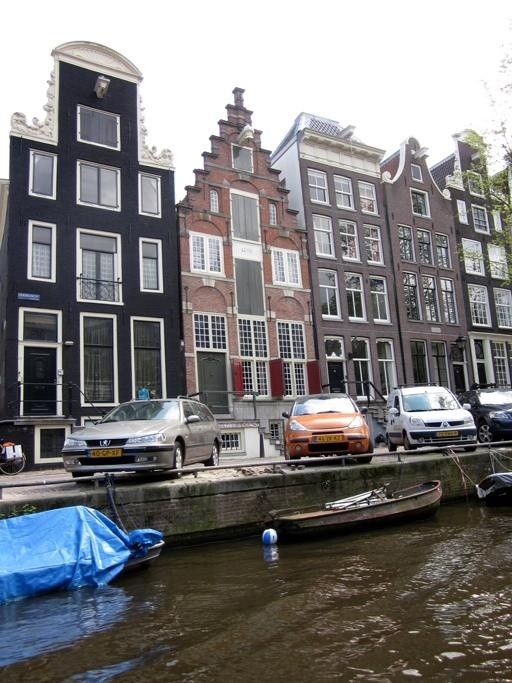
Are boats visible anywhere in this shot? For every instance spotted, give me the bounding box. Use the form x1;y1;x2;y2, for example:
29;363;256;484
264;478;445;538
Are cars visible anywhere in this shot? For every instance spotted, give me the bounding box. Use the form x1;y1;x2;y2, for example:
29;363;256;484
385;385;476;454
282;390;374;466
455;384;511;444
59;397;224;483
